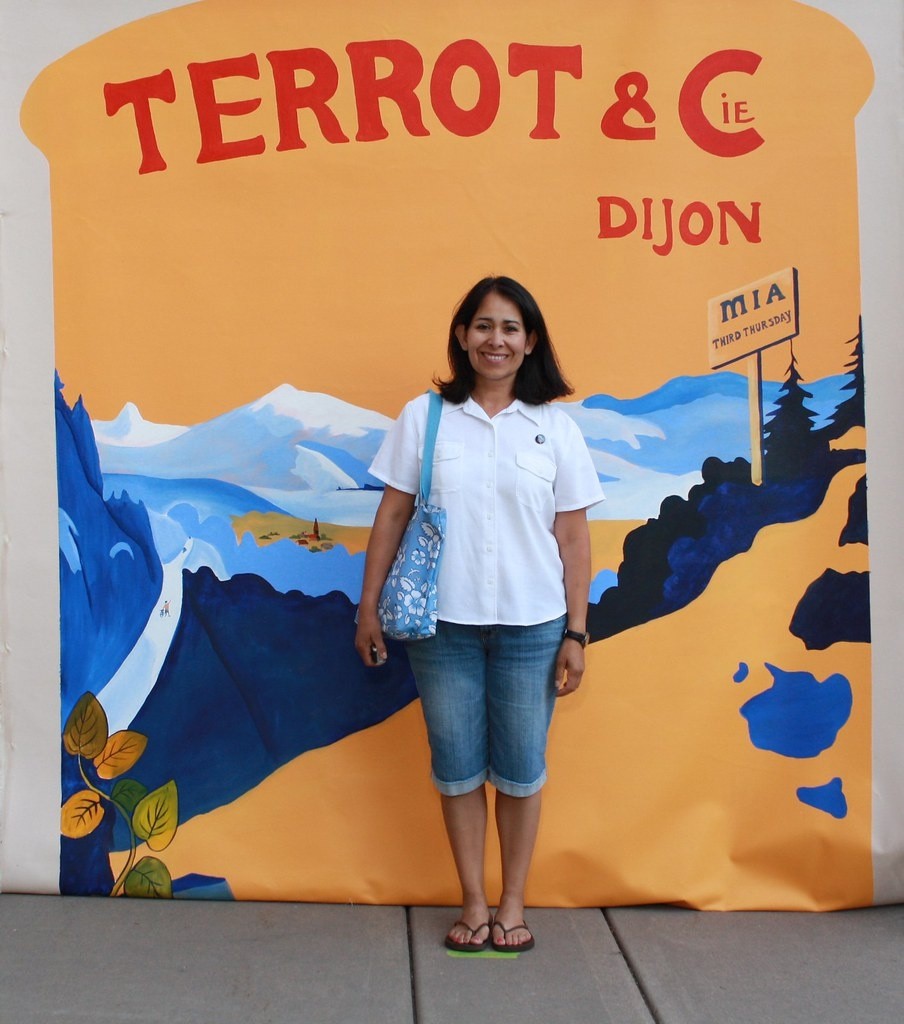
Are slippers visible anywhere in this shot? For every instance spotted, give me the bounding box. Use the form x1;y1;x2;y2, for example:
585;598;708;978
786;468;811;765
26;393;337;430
491;919;535;952
445;910;493;952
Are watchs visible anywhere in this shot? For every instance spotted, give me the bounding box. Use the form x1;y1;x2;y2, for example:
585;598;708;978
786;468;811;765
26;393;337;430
562;626;590;649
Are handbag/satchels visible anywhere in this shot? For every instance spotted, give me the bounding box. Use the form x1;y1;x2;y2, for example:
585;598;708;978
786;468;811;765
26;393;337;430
353;389;449;643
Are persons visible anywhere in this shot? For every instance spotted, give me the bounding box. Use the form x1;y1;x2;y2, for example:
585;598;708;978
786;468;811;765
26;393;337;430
355;274;607;955
